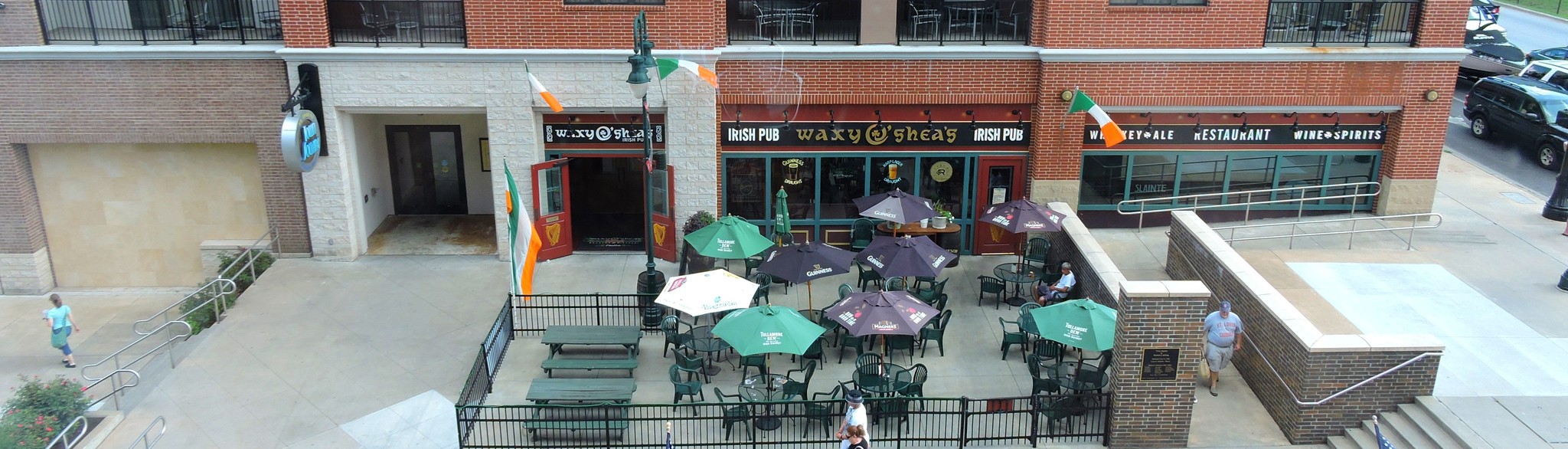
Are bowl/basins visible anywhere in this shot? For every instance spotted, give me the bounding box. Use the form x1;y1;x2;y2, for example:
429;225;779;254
887;219;901;229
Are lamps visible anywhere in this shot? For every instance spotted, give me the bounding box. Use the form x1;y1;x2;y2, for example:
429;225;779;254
563;88;1439;138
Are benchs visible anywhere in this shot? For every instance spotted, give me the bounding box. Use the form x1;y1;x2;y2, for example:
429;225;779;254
541;358;638;370
522;418;628;430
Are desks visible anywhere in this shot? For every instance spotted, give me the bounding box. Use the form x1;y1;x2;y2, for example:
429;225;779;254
1318;20;1347;41
944;0;998;38
682;325;735;376
759;0;812;38
993;262;1044;311
759;242;795;284
395;21;421;41
853;363;912;417
738;373;798;438
220;21;242;39
527;325;641;441
876;221;960;242
1047;361;1110;424
1017;313;1041;350
795;309;838;363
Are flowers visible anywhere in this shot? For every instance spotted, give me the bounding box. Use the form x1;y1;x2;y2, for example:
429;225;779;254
933;199;955;224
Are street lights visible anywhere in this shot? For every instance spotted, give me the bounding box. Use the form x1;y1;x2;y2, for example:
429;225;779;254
626;10;676;327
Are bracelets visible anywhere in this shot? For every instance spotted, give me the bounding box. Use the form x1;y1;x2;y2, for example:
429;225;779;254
840;435;843;440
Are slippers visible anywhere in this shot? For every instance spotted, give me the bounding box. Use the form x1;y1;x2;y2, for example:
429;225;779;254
62;359;68;363
66;362;76;368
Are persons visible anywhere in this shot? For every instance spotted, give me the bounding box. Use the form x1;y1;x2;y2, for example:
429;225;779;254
846;425;869;449
835;389;869;449
992;169;1003;186
43;293;78;368
1033;262;1076;307
1200;301;1245;396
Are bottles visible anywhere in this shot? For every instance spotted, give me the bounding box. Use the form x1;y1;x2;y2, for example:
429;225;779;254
1067;364;1075;376
1012;263;1017;273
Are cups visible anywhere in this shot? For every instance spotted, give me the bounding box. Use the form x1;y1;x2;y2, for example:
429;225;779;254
889;164;897;179
920;218;928;228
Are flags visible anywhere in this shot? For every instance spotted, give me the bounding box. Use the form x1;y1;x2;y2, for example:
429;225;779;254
655;58;718;88
1067;90;1127;148
525;65;564;112
504;160;542;301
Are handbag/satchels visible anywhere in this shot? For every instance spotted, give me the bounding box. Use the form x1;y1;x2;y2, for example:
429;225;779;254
1198;345;1211;379
51;327;67;347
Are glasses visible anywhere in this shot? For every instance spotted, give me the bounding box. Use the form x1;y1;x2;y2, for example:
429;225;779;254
845;433;854;439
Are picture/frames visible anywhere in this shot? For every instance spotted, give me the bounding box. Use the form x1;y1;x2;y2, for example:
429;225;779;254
479;137;491;172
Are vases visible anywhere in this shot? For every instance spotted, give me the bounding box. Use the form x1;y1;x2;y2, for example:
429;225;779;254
931;216;947;229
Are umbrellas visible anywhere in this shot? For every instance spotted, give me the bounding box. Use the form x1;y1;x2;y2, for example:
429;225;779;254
654;269;760;369
773;185;791;247
852;187;940;238
856;235;957;290
682;212;775;271
978;196;1067;271
1029;296;1118;371
756;240;858;320
711;303;827;420
824;289;940;378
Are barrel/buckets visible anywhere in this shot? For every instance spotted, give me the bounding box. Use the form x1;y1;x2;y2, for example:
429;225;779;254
936;229;961;268
637;271;666;315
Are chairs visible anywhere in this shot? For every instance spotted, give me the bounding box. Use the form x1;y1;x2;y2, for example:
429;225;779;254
1267;0;1387;42
358;1;464;40
751;0;1027;41
663;217;1112;442
836;158;962;215
166;0;281;40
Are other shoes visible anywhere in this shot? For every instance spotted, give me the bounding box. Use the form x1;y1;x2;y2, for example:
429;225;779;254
1216;377;1219;382
1209;385;1218;396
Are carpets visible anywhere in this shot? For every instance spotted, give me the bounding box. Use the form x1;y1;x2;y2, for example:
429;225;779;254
576;212;646;251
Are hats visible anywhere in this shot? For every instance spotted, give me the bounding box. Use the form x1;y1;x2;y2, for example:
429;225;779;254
1220;301;1230;312
845;390;864;404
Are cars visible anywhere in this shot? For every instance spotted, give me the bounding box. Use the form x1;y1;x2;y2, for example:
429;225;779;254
1464;0;1508;45
1462;45;1568;170
